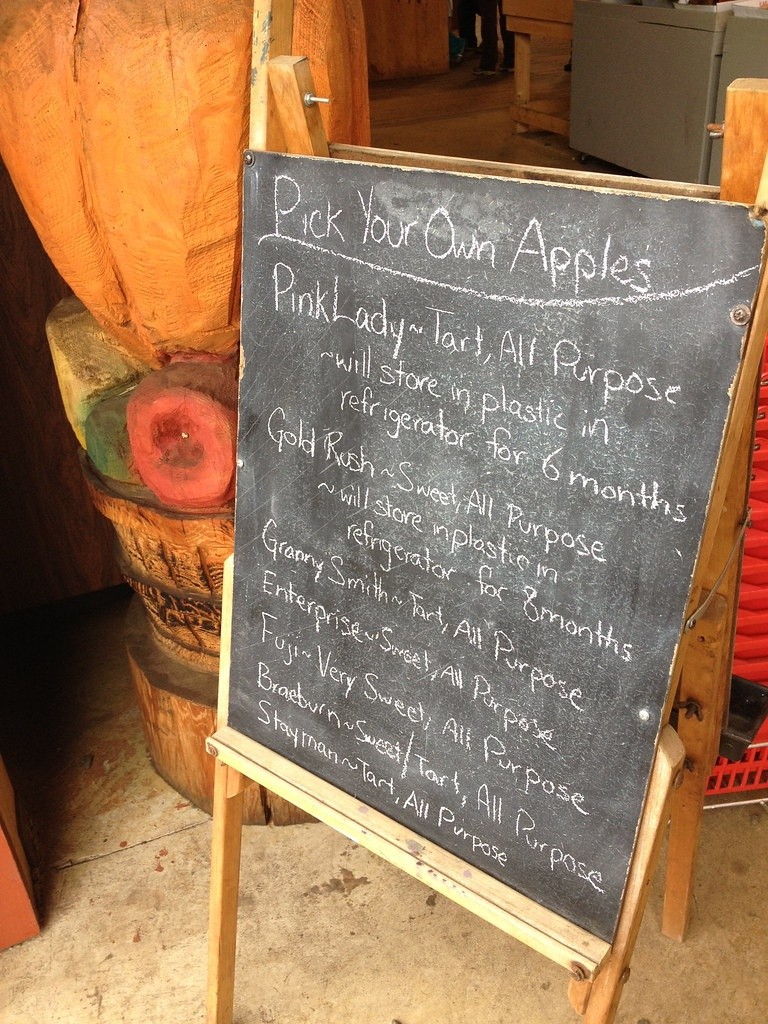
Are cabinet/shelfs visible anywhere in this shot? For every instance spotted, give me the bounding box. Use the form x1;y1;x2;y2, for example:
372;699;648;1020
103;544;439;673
502;0;768;185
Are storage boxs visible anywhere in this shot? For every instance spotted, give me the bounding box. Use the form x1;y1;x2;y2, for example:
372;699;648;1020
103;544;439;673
703;337;767;795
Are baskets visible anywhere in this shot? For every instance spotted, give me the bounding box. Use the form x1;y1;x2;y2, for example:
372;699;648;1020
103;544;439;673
704;339;767;797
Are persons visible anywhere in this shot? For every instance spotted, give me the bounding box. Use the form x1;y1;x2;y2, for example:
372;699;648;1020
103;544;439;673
472;0;516;76
456;0;481;49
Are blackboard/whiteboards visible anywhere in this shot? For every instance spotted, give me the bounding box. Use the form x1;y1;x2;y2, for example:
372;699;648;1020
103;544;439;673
212;145;768;975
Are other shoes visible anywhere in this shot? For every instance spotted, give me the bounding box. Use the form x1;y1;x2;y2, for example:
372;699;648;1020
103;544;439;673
500;63;514;72
472;66;496;76
